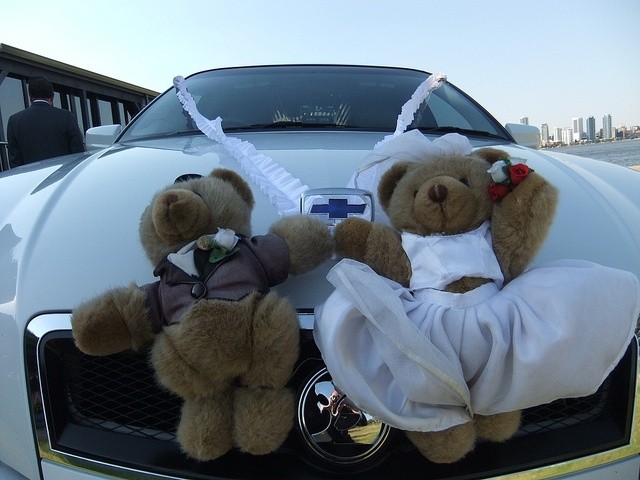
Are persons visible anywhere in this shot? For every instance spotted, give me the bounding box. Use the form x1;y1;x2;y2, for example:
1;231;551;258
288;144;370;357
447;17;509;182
7;75;84;170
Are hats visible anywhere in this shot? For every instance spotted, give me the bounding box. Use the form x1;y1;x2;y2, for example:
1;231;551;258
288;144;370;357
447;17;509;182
29;76;53;98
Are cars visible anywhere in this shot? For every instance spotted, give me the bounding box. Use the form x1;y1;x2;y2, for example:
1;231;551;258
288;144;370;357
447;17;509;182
0;63;640;480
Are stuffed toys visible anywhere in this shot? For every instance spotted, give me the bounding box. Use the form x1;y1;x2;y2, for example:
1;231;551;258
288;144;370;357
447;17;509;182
312;149;640;464
70;169;336;462
